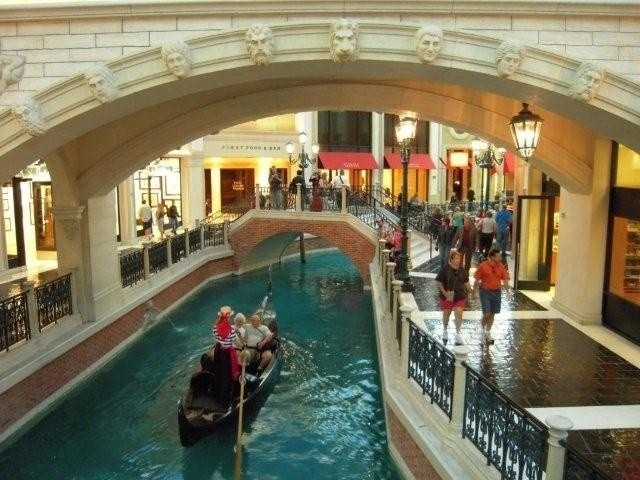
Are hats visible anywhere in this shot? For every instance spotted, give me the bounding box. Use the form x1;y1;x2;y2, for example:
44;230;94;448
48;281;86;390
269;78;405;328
217;306;235;317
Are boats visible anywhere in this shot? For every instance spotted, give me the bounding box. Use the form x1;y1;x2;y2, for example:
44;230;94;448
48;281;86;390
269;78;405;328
177;280;283;448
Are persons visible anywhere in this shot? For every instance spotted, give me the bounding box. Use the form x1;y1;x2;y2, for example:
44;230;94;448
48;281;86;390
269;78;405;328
387;226;402;263
139;199;153;236
156;203;166;240
10;93;48;135
167;199;181;236
568;61;607;105
472;249;511;345
244;21;276;67
493;38;524;80
258;165;351;210
160;38;194;81
187;300;275;414
83;64;119;106
409;188;514;278
377;216;392;249
433;250;472;347
414;25;443;64
325;16;360;64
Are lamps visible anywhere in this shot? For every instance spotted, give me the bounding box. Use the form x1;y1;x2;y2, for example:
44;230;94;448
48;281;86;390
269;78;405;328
506;101;545;165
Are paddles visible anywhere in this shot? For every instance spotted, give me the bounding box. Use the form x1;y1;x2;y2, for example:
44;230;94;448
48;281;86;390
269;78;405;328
234;341;247;480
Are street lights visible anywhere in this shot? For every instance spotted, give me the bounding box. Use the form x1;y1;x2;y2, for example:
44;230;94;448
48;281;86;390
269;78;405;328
471;137;506;216
286;130;320;178
394;111;418;292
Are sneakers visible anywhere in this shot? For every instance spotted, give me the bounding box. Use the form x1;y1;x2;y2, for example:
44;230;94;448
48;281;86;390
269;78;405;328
442;330;495;344
